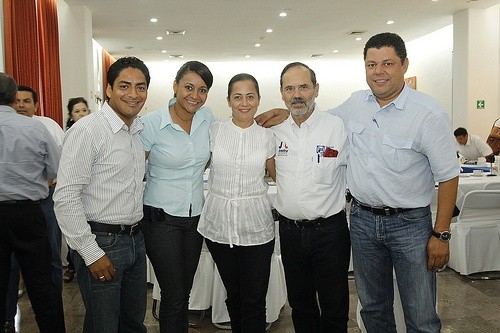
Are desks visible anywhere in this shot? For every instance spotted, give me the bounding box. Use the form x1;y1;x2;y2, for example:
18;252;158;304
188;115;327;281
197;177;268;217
429;172;500;226
146;166;290;284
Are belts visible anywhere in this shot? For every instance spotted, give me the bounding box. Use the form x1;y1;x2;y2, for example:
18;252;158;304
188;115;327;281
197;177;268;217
88;220;139;236
283;216;317;229
352;198;409;217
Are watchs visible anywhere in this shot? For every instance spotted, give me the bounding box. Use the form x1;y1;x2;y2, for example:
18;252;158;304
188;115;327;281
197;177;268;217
432;230;452;242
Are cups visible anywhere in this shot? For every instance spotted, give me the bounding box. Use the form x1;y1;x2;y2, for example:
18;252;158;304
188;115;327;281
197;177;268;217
473;170;483;175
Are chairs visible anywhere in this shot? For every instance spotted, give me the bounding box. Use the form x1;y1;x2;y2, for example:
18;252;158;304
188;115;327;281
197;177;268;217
447;183;500;276
152;190;287;332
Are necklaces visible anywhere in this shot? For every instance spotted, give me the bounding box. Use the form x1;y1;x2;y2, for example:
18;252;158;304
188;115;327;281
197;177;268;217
173;102;193;121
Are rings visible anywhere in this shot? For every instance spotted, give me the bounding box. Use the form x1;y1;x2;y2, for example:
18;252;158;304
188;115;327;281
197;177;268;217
99;276;105;279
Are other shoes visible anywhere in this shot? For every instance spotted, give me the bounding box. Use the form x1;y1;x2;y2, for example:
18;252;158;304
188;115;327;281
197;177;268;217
63;267;74;282
5;319;15;333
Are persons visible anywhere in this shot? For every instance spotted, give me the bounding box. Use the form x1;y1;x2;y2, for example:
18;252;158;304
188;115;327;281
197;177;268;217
53;57;151;333
488;128;500;156
0;72;91;333
197;73;281;333
267;62;349;333
140;61;288;333
454;127;495;163
255;33;459;333
